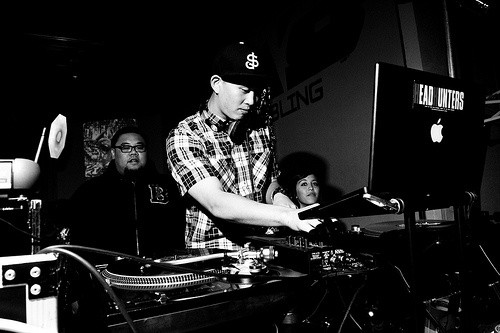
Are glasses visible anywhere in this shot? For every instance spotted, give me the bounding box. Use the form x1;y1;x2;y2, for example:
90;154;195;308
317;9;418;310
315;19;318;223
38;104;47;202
113;144;146;153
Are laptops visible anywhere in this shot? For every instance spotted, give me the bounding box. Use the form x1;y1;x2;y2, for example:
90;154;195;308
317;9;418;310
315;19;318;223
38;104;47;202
298;61;484;220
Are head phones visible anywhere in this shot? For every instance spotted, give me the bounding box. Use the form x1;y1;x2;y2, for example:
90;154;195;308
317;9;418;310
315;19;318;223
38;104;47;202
201;102;248;145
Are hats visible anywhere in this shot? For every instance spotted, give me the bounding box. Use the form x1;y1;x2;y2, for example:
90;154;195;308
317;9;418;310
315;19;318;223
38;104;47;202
212;41;272;88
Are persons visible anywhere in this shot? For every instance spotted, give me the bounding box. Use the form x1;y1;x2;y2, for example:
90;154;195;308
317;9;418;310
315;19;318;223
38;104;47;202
283;162;320;210
69;128;182;263
166;43;338;250
